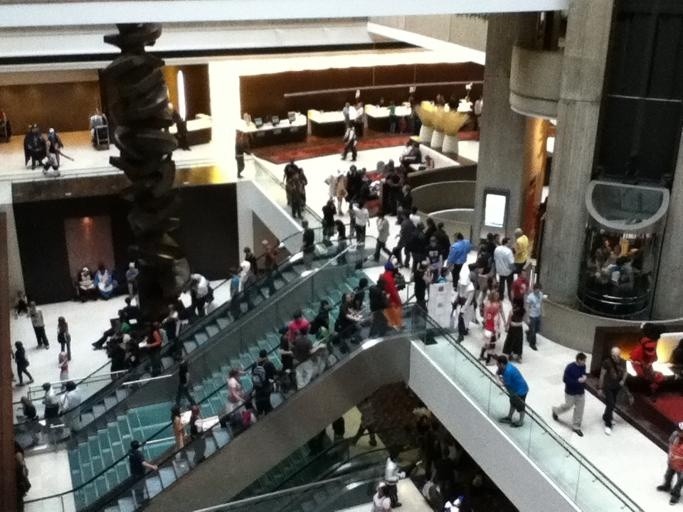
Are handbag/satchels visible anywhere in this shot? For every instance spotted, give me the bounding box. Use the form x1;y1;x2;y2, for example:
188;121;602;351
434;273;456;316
615;386;634;407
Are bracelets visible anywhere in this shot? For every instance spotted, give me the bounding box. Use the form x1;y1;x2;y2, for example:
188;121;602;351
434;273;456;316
596;387;604;390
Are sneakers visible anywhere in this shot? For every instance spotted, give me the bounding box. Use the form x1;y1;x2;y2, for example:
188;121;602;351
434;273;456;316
499;412;615;437
670;496;677;503
454;320;538;366
657;486;668;491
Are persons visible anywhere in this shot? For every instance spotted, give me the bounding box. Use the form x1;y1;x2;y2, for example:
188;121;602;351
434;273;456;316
584;233;655;296
308;394;514;512
321;143;471;345
280;155;308;219
495;354;530;428
655;420;683;505
469;226;546;368
92;215;335;505
337;93;484;162
0;110;12;143
23;122;63;178
551;352;588;437
669;337;683;382
596;345;629;436
630;321;666;394
234;137;251;179
8;266;95;512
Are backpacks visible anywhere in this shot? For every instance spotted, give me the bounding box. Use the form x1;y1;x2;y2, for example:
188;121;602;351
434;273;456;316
251;361;265;388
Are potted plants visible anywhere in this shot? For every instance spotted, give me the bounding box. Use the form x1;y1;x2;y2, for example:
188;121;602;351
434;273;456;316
413;100;470;160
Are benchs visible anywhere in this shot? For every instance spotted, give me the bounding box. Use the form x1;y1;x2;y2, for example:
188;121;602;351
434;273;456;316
399;144;460;171
